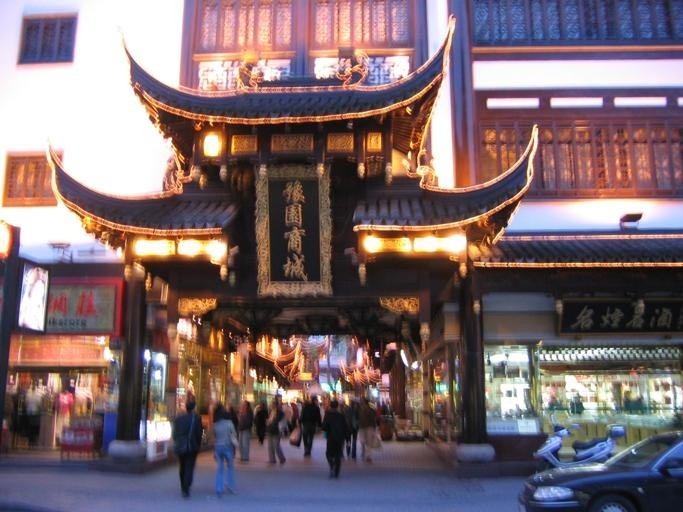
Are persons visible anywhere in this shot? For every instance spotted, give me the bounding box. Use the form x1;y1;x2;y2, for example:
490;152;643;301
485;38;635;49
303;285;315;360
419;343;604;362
621;390;647;414
24;377;75;449
171;391;394;499
549;395;585;415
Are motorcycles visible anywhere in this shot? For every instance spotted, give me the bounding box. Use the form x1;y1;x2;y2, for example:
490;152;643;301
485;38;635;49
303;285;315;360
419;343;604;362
532;414;626;471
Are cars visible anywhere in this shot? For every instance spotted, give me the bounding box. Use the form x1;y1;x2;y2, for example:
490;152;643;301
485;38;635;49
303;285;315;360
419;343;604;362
519;430;683;511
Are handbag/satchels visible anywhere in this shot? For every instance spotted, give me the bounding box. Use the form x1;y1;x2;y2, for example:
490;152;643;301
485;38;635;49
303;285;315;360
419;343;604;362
324;432;327;438
231;434;240;448
371;432;380;448
173;438;192;455
289;426;302;446
